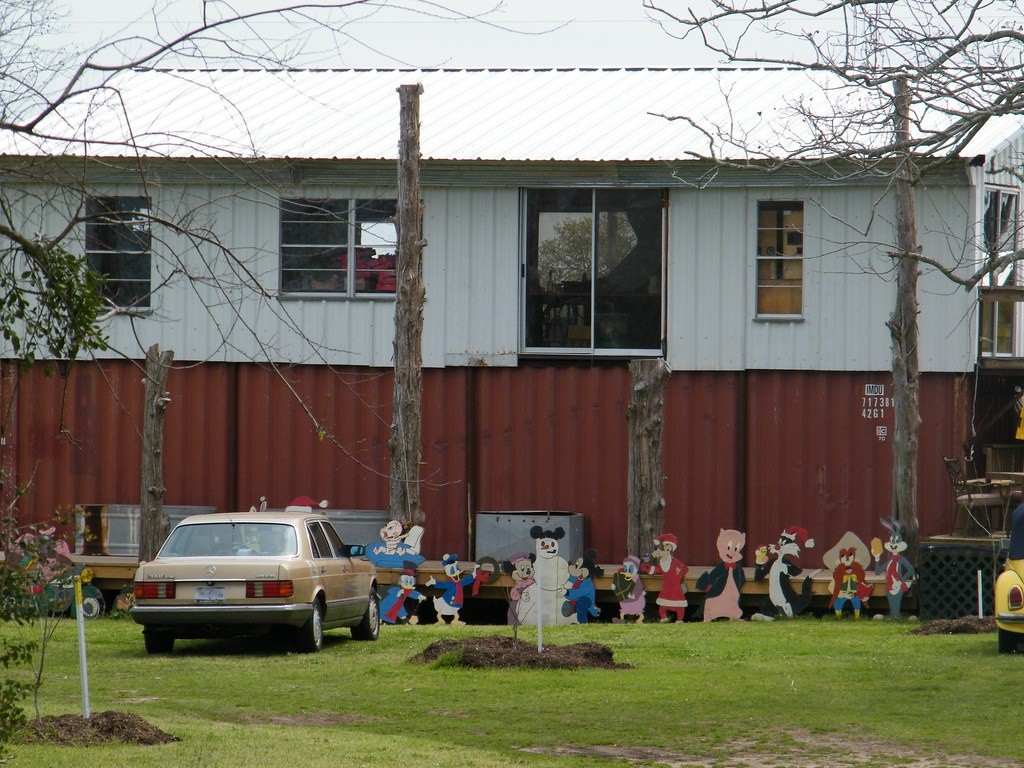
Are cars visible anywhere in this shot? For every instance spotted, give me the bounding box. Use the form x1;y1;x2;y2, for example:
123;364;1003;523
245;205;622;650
994;498;1024;653
130;511;379;652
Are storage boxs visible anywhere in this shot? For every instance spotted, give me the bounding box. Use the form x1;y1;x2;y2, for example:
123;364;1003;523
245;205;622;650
758;210;777;280
476;509;583;565
783;210;803;279
758;280;802;315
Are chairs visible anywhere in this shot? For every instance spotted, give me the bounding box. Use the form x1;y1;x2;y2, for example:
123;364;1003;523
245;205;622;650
942;454;1009;538
